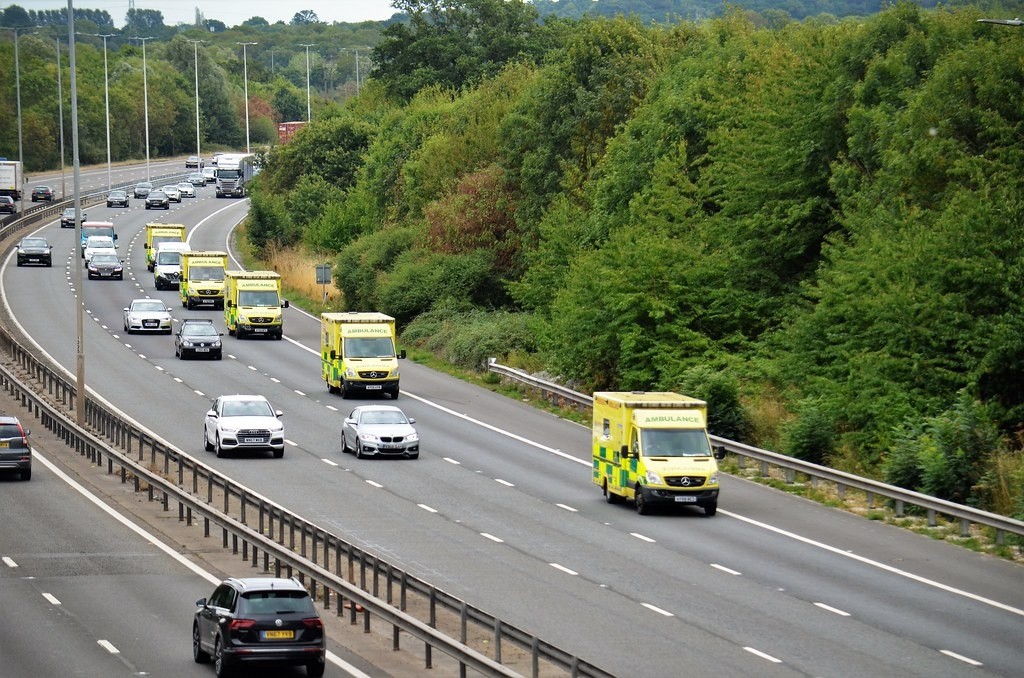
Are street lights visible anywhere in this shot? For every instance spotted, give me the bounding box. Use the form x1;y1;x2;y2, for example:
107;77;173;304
342;47;371;97
47;33;70;204
0;25;43;220
235;41;260;153
126;36;160;183
299;43;317;125
75;31;120;193
184;38;209;174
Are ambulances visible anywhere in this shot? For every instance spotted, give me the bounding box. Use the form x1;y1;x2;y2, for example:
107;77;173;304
320;311;407;400
144;222;187;273
590;392;727;517
180;251;229;311
224;270;290;341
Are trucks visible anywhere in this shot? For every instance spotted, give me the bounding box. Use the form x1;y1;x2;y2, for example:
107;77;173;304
277;122;306;145
215;154;255;199
0;161;29;200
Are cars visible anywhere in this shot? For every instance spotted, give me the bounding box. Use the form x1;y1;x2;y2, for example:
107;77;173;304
82;236;120;269
87;251;125;280
32;186;56;203
186;156;205;168
133;182;154;198
187;173;207;187
159;186;182;203
211;153;224;164
59;208;88;228
106;191;130;208
201;167;217;183
122;299;174;335
177;183;196;198
339;403;421;460
173;319;224;360
145;191;170;210
0;196;17;215
15;237;53;267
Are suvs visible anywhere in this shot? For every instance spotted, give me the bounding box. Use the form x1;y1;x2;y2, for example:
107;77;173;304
202;394;286;458
191;577;325;678
0;417;35;481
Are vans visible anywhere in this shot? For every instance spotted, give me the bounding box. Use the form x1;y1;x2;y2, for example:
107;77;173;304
80;222;118;258
154;241;191;292
